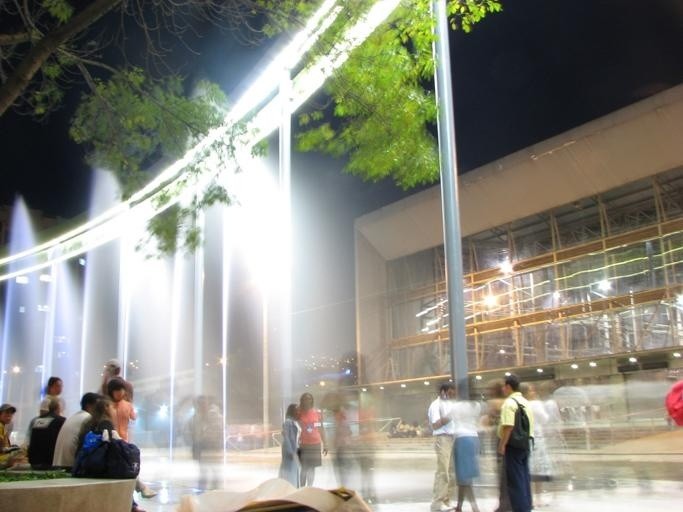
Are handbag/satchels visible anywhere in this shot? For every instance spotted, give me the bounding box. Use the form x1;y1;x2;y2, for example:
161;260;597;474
83;437;140;476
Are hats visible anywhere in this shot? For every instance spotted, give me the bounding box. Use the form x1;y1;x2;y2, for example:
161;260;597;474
0;404;16;413
107;359;120;369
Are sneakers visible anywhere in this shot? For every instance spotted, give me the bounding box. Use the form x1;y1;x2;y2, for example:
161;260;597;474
141;489;157;498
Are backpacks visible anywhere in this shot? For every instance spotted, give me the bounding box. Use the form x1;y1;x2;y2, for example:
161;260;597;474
509;395;530;451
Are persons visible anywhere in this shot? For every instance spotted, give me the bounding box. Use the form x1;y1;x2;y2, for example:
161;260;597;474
278;392;328;488
1;360;158;511
427;373;485;511
479;374;554;511
387;418;433;439
182;394;225;494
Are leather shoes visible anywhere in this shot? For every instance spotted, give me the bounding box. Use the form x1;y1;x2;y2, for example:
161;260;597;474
431;503;454;512
